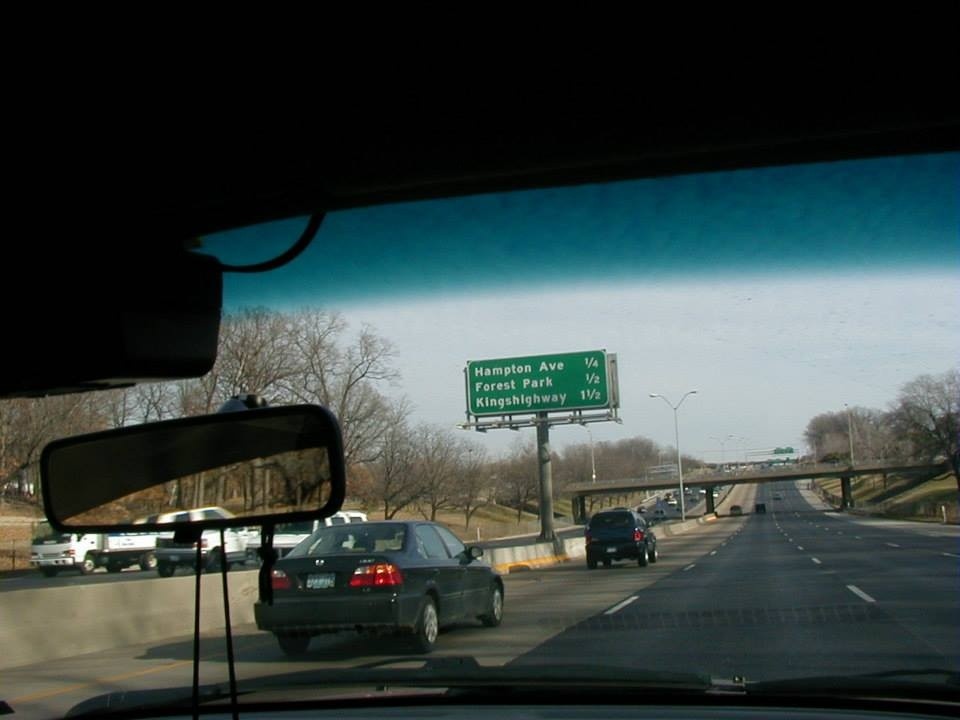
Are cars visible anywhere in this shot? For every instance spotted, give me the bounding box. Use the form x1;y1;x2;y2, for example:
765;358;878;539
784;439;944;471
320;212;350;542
685;489;692;495
668;498;677;504
712;491;718;497
665;493;672;499
699;489;706;495
254;520;504;653
774;491;782;499
730;505;742;515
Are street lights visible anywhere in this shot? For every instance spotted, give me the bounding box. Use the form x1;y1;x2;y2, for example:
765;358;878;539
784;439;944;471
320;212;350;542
649;390;698;522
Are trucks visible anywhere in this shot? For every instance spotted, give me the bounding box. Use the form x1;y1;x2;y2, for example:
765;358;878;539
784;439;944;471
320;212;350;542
29;518;158;576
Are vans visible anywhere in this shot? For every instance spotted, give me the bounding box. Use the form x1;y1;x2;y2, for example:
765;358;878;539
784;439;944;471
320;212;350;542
654;509;665;518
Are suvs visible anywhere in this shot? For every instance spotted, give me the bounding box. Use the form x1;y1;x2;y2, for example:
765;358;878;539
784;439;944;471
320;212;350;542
585;507;658;569
153;525;248;578
756;503;765;512
132;506;367;558
